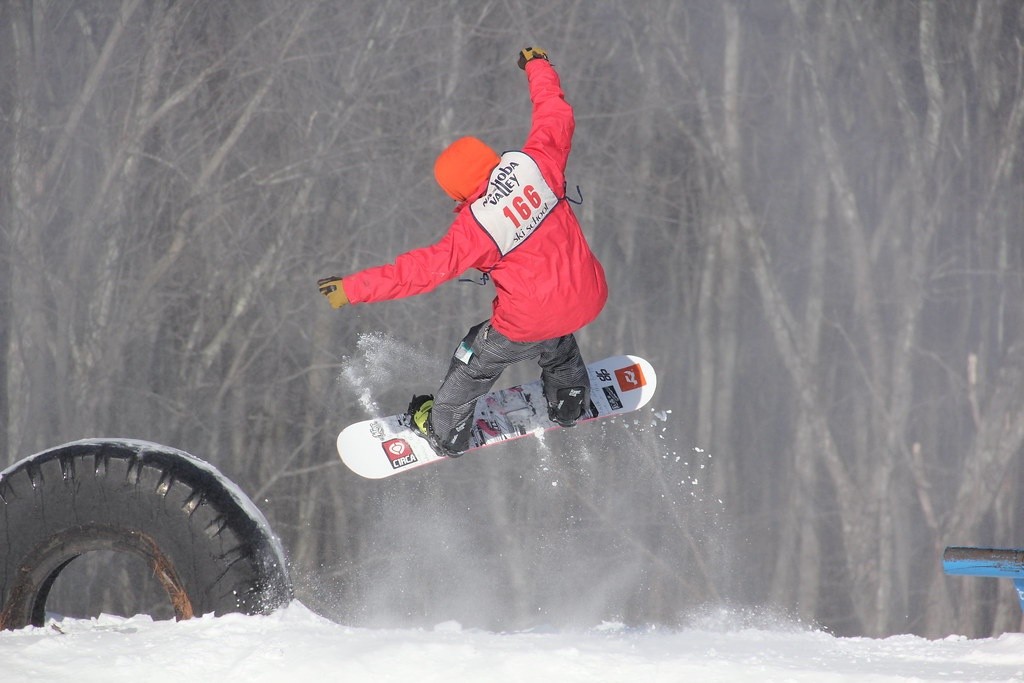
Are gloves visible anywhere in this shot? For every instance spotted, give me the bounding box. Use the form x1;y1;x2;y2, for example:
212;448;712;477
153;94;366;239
517;47;548;69
316;276;347;308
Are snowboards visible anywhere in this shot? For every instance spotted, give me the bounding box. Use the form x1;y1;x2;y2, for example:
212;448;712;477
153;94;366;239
335;354;658;481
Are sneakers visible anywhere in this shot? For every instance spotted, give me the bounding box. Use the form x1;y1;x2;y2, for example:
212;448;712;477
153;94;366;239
540;369;584;427
413;393;463;458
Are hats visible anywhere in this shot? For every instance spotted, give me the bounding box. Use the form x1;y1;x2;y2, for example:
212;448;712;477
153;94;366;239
434;137;500;202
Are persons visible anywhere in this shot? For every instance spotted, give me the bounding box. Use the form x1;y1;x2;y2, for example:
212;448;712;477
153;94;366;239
317;48;609;457
